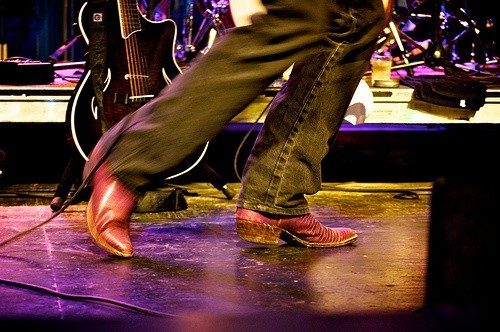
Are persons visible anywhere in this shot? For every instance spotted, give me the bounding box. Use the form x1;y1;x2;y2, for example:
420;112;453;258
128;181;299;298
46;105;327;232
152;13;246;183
82;0;385;258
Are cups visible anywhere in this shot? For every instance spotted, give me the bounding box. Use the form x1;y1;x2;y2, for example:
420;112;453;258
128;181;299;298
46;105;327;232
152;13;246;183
372;55;392;86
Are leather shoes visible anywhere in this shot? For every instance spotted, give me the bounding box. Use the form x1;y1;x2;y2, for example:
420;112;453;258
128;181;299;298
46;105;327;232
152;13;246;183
84;139;137;257
236;207;358;247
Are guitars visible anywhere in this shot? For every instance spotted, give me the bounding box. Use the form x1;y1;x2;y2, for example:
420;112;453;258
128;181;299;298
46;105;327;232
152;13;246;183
65;0;209;180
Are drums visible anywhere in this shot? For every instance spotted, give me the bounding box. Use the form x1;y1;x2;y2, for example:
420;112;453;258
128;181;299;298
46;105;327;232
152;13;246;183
212;0;267;32
